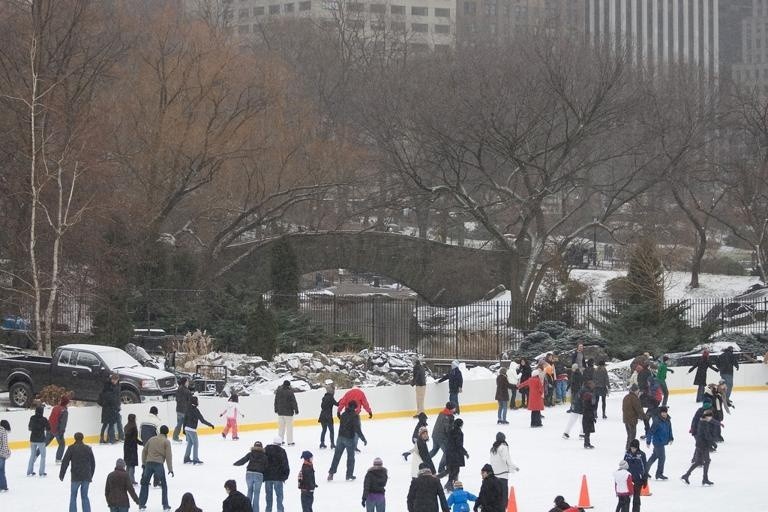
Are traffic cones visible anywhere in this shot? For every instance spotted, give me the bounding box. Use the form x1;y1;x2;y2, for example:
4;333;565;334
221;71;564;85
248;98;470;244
637;475;652;497
506;487;519;512
575;473;596;509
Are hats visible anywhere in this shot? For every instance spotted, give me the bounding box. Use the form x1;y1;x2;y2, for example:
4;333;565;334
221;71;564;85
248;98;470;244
452;360;459;367
61;396;70;406
418;401;505;442
619;460;629;470
374;457;493;489
632;383;638;391
301;451;313;460
532;370;539;375
355;382;361;387
255;442;262;448
150;407;158;414
581;392;593;401
160;425;168;434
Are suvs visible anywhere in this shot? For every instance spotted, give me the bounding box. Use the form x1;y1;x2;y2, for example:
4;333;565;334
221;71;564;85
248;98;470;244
657;343;745;366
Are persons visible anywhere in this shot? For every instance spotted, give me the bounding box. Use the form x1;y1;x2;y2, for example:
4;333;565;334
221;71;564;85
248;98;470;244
621;344;739;485
493;343;611;449
0;420;11;492
27;406;51;476
336;389;373;420
298;451;318;512
274;380;298;446
222;480;254;511
326;399;356;481
549;438;650;512
233;442;268;512
403;357;521;512
36;396;71;464
317;384;339;449
219;393;244;440
361;457;388;512
97;372;214;512
262;442;289;511
59;432;95;512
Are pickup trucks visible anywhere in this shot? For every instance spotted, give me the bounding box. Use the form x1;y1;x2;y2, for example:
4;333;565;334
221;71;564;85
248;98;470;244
0;343;180;404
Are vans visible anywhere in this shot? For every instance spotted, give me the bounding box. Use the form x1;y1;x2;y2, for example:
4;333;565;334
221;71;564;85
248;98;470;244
132;328;169;342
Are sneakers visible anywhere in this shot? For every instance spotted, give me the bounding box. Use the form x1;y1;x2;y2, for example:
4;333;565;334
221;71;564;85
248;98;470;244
320;442;360;480
100;436;203;510
562;415;607;448
648;473;713;484
233;437;239;439
0;460;64;491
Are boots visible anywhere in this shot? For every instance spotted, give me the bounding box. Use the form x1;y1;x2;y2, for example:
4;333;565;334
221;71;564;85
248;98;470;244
402;452;411;461
